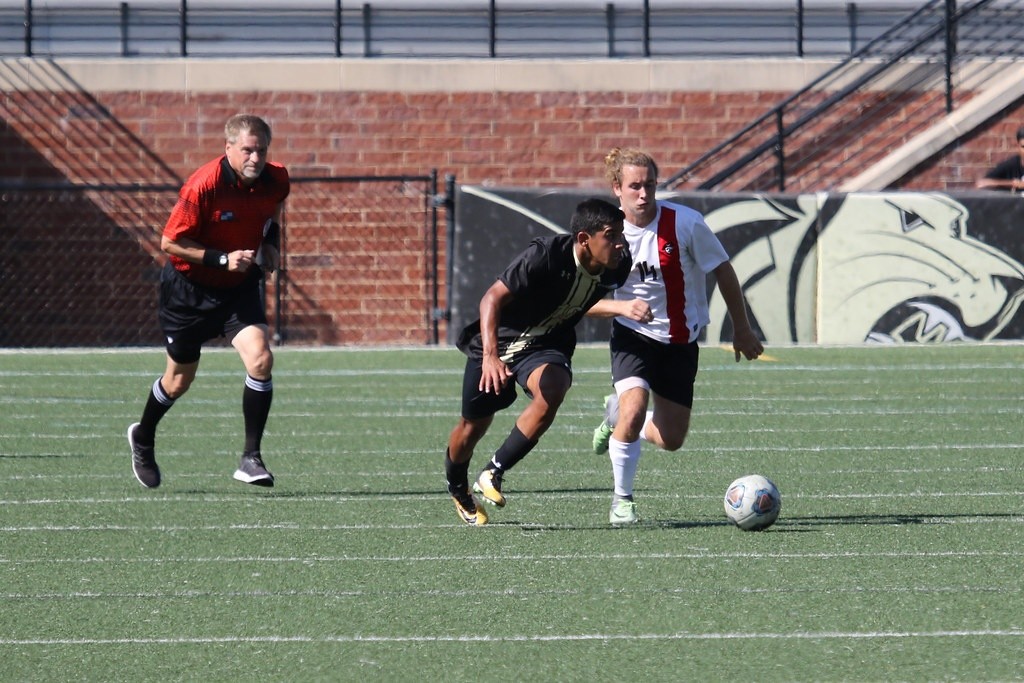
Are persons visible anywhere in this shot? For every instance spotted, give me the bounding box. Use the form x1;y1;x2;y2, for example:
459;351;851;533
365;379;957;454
584;146;764;527
445;198;633;526
127;114;291;490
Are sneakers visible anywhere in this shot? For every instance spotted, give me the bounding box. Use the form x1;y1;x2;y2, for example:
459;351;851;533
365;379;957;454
471;468;506;509
446;481;489;524
232;455;274;487
609;498;639;527
126;422;162;487
592;394;616;456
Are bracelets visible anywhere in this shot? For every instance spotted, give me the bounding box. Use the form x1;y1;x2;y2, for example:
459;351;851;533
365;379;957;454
202;249;228;271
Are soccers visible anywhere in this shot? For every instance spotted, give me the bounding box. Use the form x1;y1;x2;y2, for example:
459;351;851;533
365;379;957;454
724;473;781;531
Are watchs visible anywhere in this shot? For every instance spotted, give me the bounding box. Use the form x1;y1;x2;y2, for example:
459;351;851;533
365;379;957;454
219;252;228;269
